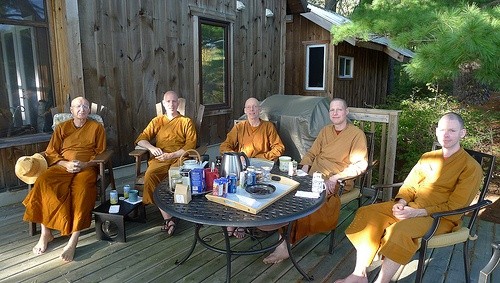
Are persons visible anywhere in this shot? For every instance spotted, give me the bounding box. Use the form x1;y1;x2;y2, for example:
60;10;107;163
220;98;285;239
135;90;198;236
332;112;482;283
22;97;107;263
263;99;368;264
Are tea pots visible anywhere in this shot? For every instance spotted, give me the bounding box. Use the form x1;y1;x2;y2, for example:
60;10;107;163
180;150;209;170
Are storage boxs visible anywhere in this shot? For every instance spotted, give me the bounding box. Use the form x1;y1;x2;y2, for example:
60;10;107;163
180;168;191;191
190;168;206;194
168;167;179;194
173;183;192;205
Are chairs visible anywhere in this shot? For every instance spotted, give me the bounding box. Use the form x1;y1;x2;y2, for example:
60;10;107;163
234;119;279;134
28;103;116;237
330;131;377;257
128;99;206;218
370;141;497;283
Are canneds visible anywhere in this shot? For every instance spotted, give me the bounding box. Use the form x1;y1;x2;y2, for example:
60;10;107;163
123;184;131;198
110;190;118;204
227;166;263;194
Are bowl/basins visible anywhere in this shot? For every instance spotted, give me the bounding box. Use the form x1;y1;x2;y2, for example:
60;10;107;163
245;158;274;172
246;183;276;198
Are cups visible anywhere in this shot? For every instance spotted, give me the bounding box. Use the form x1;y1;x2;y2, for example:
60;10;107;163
205;168;220;188
128;190;138;202
279;156;291;171
311;172;327;193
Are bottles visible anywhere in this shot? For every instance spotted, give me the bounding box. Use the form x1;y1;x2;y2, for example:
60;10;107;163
216;155;222;170
170;175;184;193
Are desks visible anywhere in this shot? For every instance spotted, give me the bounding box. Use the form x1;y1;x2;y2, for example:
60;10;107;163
152;163;326;283
91;194;147;243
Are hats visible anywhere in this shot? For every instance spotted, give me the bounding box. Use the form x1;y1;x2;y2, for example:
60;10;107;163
15;153;49;185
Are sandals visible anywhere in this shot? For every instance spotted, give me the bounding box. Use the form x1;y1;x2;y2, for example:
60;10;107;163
222;227;246;239
160;216;177;236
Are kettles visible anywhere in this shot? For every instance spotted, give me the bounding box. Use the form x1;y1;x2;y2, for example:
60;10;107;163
220;151;250;182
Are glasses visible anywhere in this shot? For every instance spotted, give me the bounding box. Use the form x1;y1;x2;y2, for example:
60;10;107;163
245;104;261;110
329;107;344;113
71;104;89;110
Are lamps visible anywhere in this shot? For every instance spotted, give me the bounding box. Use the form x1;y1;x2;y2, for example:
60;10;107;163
265;8;274;18
235;1;246;11
